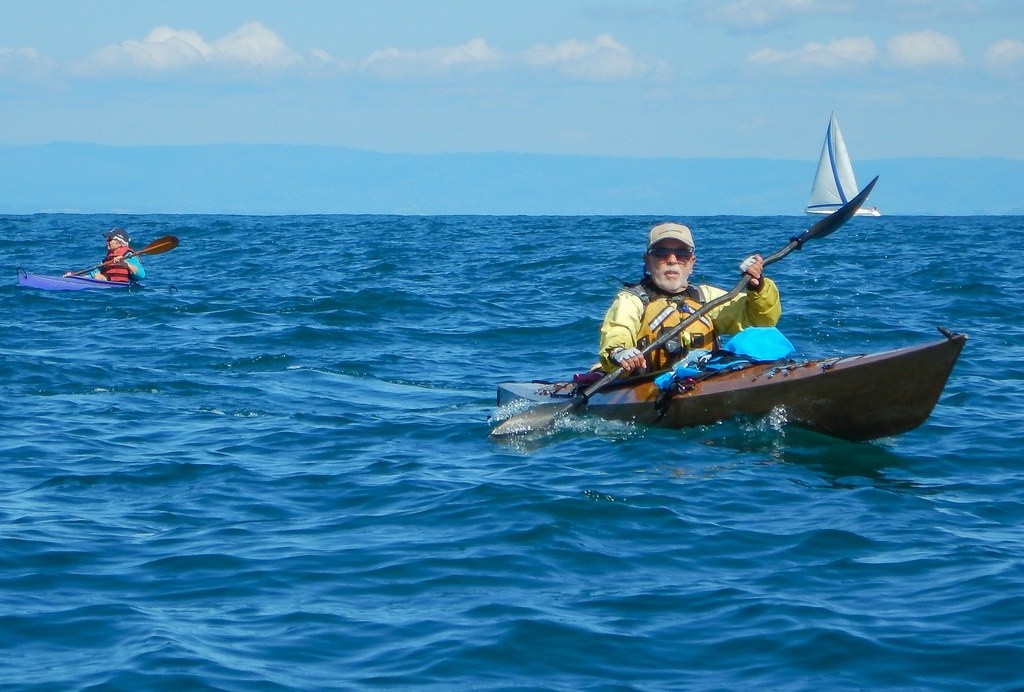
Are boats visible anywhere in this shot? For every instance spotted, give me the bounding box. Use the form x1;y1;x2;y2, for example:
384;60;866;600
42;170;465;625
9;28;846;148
494;324;970;443
16;266;180;296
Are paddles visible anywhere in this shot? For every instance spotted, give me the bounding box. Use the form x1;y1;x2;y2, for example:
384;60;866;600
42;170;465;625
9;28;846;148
489;172;887;439
64;235;181;278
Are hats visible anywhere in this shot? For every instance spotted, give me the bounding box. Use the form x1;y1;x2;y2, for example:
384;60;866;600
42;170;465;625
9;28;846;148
102;229;132;242
649;223;695;248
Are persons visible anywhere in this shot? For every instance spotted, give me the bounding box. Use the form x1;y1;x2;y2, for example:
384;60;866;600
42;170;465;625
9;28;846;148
598;223;783;381
63;228;146;283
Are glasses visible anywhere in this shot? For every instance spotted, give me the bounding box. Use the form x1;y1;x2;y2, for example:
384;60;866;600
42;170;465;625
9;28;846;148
107;238;118;242
646;246;691;259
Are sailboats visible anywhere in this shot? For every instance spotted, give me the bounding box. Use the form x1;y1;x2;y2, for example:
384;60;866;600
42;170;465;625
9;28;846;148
801;106;883;216
